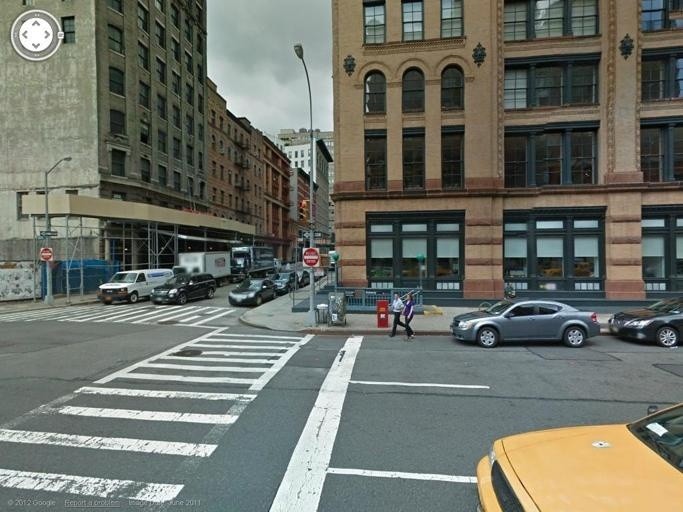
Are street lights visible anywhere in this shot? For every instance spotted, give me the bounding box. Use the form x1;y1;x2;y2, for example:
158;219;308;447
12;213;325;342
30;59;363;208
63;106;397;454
41;154;75;305
292;40;325;331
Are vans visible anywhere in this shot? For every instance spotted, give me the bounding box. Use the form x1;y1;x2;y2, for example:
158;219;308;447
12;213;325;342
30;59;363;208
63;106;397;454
96;267;175;305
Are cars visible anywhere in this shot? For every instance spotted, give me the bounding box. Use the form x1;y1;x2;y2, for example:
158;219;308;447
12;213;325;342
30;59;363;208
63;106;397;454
477;398;682;511
267;267;320;295
447;296;604;349
228;277;278;307
606;296;683;348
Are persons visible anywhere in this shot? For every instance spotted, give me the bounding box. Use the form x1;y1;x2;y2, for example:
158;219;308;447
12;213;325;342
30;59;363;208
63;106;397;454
403;293;414;339
389;291;406;337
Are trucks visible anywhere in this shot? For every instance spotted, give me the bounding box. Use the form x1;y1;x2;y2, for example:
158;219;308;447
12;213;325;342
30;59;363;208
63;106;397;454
170;249;232;289
228;246;275;282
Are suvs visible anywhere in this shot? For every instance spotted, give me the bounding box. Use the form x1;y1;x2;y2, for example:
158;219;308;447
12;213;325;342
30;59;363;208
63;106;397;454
150;272;218;307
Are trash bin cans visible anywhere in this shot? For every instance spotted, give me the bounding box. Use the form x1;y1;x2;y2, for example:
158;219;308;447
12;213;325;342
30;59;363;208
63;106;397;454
316;304;328;323
